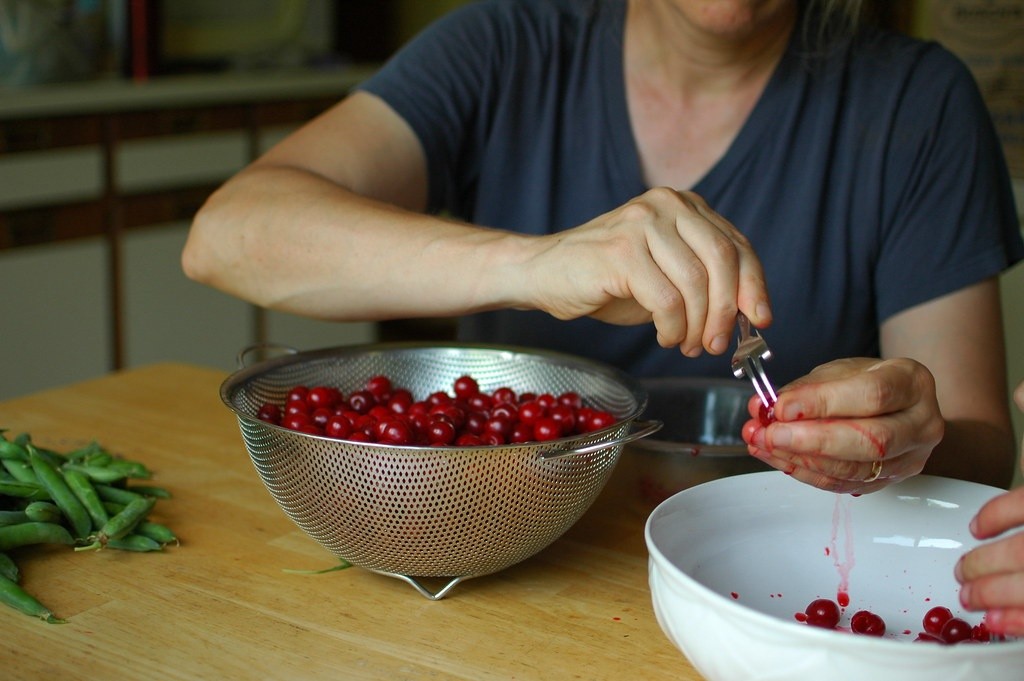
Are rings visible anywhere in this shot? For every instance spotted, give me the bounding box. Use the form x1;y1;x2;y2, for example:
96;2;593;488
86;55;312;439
862;460;884;483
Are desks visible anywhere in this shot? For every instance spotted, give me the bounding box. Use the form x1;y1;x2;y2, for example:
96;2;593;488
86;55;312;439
1;360;746;680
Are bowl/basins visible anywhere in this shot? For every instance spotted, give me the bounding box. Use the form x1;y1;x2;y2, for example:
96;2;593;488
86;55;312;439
622;376;777;520
643;471;1024;681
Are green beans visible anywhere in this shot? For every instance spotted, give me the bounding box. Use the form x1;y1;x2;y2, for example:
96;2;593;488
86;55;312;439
0;424;178;623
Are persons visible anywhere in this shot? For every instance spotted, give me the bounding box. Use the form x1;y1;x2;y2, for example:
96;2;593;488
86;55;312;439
181;0;1024;494
954;381;1024;640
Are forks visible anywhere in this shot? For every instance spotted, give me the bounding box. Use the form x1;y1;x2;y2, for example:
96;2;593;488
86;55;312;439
734;311;780;409
